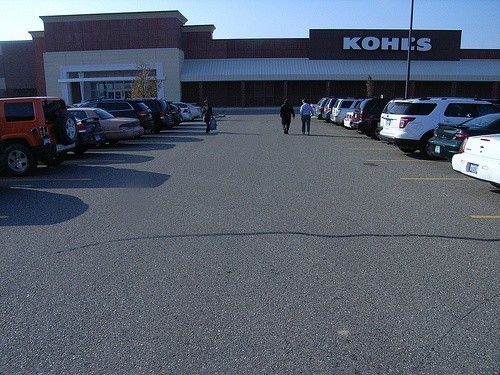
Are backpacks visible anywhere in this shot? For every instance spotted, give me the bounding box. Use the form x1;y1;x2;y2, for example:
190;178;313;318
209;117;216;130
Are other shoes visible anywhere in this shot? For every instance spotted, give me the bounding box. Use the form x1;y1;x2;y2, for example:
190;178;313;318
307;132;309;135
206;129;210;133
284;128;288;134
302;132;305;135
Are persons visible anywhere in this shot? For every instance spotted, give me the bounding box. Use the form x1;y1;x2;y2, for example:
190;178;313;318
300;99;314;136
280;99;295;135
200;98;214;133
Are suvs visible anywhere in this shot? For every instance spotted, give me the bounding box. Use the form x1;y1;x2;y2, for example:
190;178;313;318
73;99;175;139
311;96;500;155
0;98;105;176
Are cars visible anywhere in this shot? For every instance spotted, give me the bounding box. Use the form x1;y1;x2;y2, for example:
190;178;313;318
68;108;145;154
427;113;500;188
168;97;201;133
